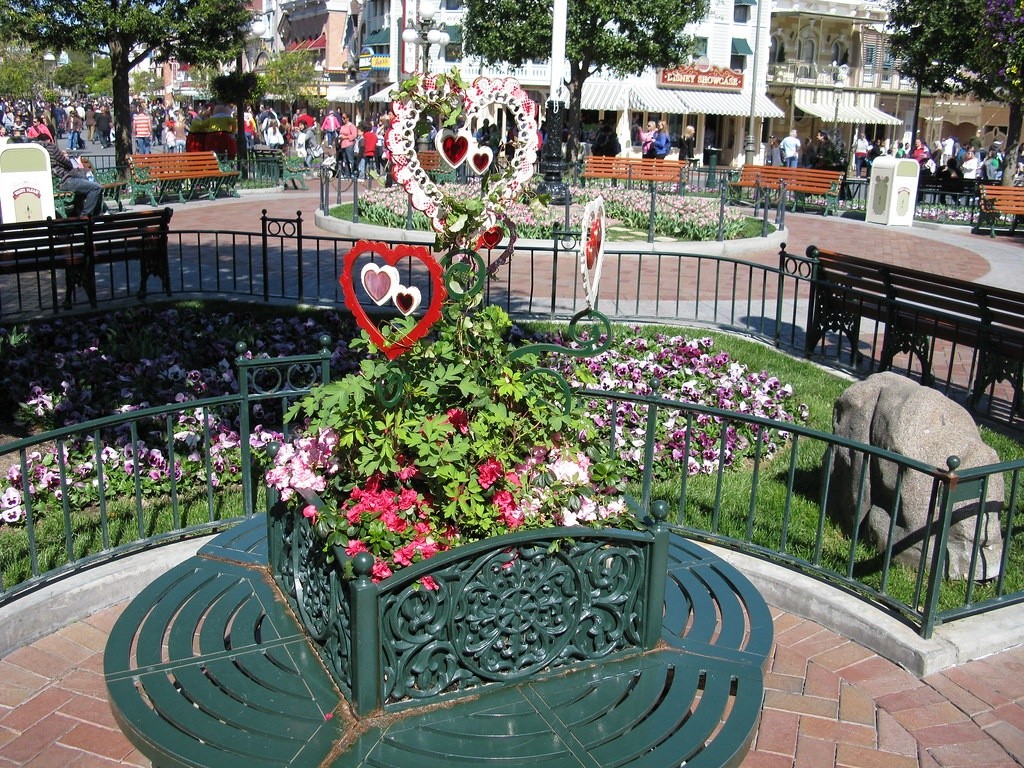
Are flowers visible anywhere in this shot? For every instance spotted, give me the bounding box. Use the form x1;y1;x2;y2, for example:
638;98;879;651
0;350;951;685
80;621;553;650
267;65;645;595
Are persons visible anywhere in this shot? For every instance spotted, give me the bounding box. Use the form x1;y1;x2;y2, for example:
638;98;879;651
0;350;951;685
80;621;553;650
631;114;695;175
852;133;1024;206
35;133;103;221
0;97;437;187
476;118;500;175
506;119;518;168
768;129;837;169
539;120;619;174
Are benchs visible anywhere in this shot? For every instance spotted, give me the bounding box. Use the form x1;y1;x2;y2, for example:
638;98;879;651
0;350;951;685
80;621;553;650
971;184;1024;238
727;164;845;217
411;150;458;185
919;175;1002;204
55;159;130;219
578;156;690;196
126;153;242;208
0;206;173;310
805;245;1024;418
251;147;311;190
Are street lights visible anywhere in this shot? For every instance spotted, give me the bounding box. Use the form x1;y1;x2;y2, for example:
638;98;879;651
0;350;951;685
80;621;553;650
43;51;57;145
402;1;450;151
221;17;266;183
150;77;156;146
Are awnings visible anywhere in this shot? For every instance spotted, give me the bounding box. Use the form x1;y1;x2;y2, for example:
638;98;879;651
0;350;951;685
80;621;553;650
735;0;757;6
368;82;400;104
794;88;904;125
177;61;192;72
732;38;754;56
327;78;368;104
441;25;463;43
361;27;390;46
545;82;785;120
358;53;390;71
263;86;327;101
284;33;325;53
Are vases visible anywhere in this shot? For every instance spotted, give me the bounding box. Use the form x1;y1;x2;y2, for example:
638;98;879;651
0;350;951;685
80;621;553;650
265;441;670;721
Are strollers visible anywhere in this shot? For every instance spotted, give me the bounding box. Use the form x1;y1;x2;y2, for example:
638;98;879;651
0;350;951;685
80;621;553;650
316;138;343;178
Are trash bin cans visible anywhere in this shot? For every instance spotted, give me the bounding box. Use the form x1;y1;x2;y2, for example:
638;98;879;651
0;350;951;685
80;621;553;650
0;143;56;251
866;156;920;225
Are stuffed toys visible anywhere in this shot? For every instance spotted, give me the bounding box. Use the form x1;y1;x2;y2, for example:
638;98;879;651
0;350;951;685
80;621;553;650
186;106;215;199
190;105;237;197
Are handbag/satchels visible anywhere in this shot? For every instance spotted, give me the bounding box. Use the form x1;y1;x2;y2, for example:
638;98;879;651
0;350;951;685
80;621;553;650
310;145;324;158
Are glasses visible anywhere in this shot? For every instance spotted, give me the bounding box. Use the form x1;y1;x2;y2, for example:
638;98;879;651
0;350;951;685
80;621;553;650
647;125;651;127
342;117;349;120
33;121;37;123
